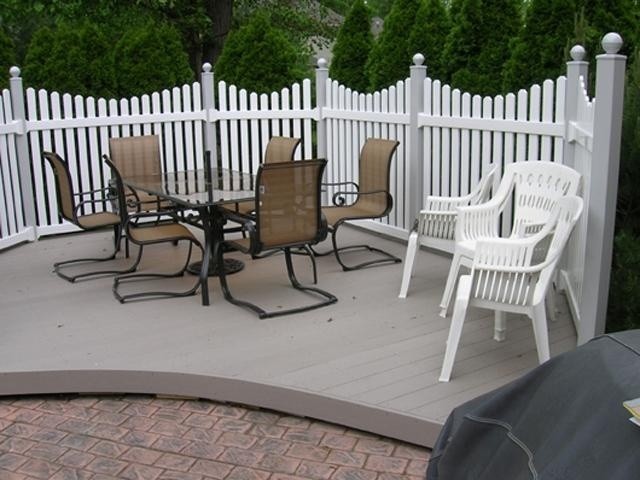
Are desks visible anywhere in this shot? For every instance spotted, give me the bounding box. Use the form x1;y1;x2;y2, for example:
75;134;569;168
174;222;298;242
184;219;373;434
122;167;262;275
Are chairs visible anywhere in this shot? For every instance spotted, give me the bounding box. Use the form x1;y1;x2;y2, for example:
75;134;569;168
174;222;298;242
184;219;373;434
103;134;182;251
320;137;403;270
399;162;499;299
215;159;339;319
439;194;584;381
242;136;303;260
42;151;121;283
101;153;205;302
439;161;582;322
